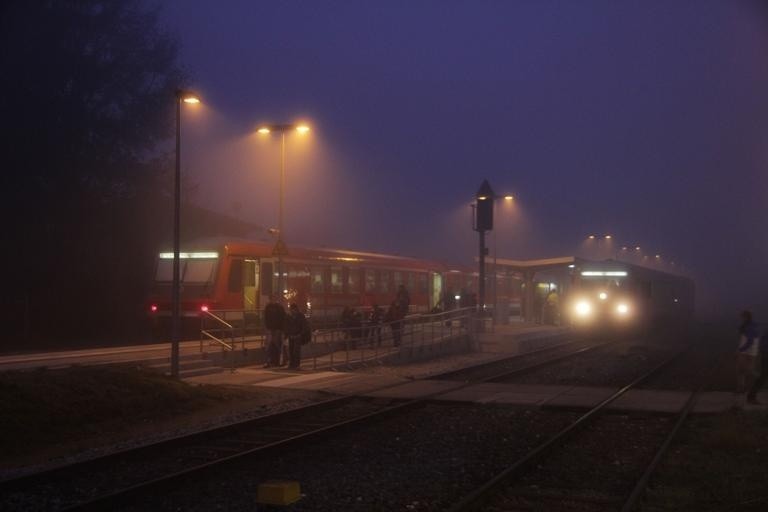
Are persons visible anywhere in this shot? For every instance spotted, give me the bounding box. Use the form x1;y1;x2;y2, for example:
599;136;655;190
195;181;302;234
746;329;768;405
257;284;564;373
733;310;759;394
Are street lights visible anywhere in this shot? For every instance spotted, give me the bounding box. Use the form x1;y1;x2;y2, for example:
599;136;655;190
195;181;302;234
480;193;516;319
256;124;311;302
171;89;203;377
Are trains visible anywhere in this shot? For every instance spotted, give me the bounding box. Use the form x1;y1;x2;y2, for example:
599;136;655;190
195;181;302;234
150;240;526;332
574;258;696;343
